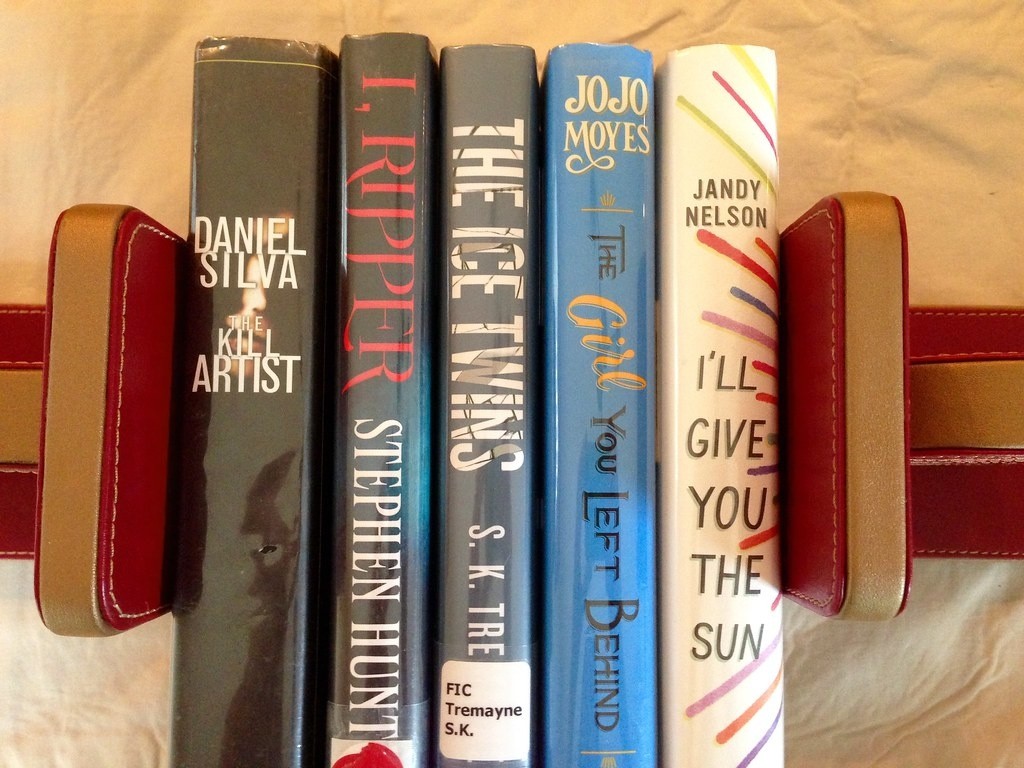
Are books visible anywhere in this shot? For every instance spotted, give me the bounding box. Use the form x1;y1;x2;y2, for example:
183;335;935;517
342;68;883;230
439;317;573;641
433;44;541;768
656;42;785;768
324;32;439;768
168;35;337;768
538;40;660;767
